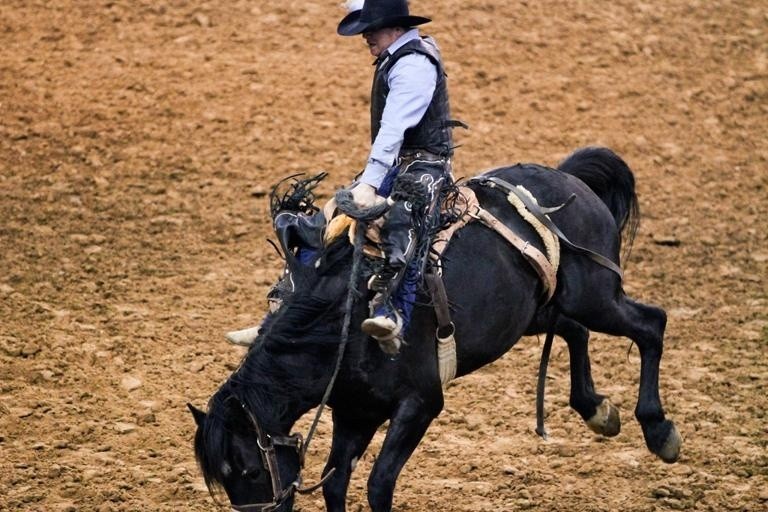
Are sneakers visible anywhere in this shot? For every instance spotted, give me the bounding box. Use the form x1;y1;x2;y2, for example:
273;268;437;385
224;326;261;347
360;315;402;355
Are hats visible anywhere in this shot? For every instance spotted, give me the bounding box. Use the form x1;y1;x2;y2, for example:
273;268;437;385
337;1;432;35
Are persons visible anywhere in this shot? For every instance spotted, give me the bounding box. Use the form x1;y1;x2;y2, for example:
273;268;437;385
226;0;454;357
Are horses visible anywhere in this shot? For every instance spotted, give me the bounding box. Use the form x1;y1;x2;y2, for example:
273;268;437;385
186;147;683;512
